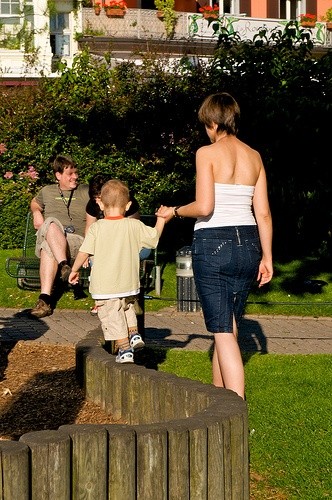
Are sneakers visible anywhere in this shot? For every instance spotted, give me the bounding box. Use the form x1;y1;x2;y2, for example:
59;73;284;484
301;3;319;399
129;333;145;352
61;264;72;281
115;347;134;364
29;299;53;318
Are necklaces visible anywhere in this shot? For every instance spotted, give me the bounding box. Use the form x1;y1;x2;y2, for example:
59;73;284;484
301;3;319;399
217;135;228;142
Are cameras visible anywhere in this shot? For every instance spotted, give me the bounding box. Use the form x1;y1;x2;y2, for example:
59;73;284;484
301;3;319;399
65;225;75;233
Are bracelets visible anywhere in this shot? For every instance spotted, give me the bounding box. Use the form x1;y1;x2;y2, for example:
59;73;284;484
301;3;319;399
173;205;185;220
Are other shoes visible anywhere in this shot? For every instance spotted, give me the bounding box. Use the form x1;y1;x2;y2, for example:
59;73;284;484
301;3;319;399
91;307;98;316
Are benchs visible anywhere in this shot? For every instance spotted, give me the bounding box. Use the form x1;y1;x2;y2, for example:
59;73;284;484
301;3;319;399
5;212;157;295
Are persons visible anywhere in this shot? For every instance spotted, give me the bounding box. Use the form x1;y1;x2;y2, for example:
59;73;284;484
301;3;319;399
155;92;274;401
27;154;90;318
66;172;169;363
